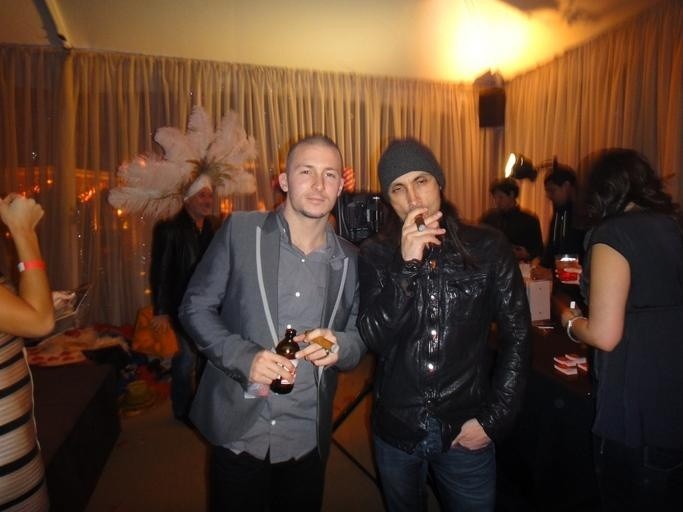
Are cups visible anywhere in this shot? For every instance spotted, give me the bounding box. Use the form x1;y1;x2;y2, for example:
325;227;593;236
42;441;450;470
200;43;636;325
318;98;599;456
554;256;579;282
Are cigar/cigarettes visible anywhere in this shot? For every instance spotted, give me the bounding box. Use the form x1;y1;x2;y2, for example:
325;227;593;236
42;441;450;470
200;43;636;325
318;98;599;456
302;329;341;355
413;212;427;235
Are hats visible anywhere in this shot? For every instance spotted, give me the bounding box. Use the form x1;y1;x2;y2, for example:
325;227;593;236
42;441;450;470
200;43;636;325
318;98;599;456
377;137;447;203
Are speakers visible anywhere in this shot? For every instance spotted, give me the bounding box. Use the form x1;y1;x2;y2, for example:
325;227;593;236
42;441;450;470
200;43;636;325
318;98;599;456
479;86;507;129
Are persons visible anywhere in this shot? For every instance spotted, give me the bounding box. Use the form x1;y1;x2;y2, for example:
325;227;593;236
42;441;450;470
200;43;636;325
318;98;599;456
476;176;544;268
178;131;370;511
147;172;223;432
552;145;683;511
353;135;533;512
0;188;76;511
529;161;591;376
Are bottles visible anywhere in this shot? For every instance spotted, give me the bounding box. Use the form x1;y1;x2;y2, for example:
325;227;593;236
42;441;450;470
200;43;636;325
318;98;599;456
268;328;301;394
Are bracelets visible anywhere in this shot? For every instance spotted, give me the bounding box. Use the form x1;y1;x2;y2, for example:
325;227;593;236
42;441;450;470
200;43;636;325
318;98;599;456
16;259;46;275
566;314;589;344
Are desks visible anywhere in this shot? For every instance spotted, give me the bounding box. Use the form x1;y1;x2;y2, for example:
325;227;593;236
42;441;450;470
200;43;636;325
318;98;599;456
489;317;599;432
